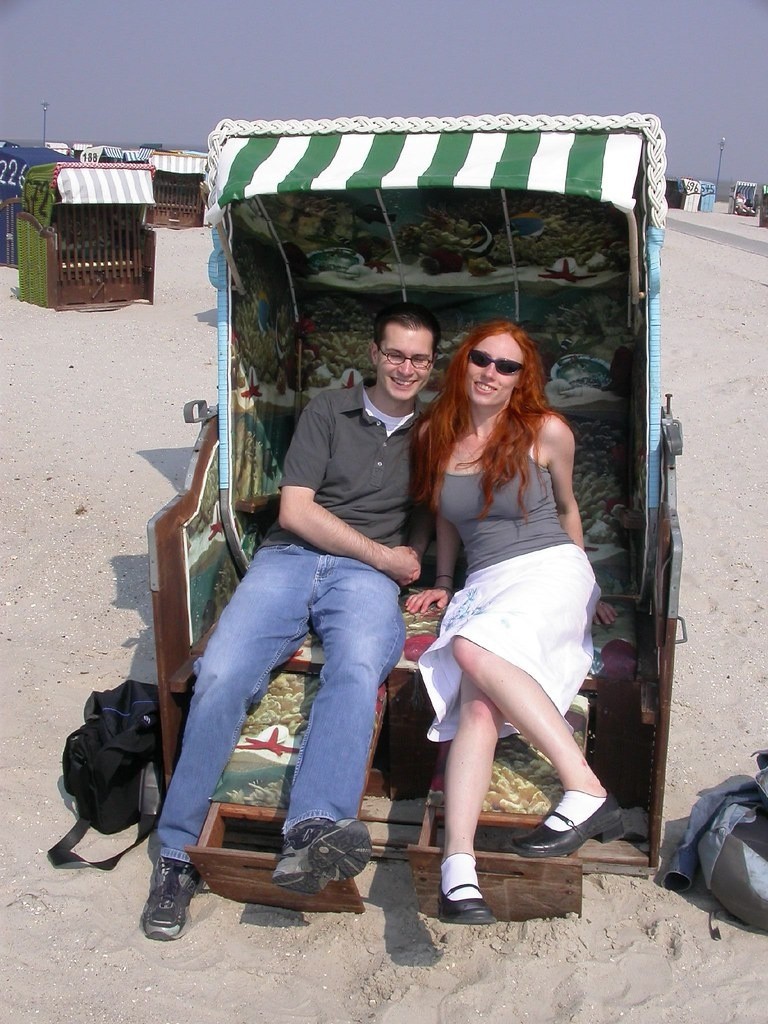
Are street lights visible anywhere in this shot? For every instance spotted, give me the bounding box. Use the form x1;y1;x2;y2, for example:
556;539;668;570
714;136;726;203
41;99;50;147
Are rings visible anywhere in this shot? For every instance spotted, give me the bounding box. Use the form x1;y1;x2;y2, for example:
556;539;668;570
600;602;605;606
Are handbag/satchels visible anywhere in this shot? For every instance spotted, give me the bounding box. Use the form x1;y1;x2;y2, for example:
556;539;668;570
62;680;167;835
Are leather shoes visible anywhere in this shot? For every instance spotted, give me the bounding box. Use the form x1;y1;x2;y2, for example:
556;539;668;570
509;788;625;857
438;877;496;924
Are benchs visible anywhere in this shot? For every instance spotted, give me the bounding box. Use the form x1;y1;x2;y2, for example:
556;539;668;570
143;392;684;925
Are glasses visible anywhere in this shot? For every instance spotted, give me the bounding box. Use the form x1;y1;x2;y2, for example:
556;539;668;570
377;346;433;369
469;350;523;375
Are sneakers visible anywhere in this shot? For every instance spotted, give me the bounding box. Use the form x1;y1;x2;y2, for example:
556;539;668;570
272;818;373;896
143;856;201;941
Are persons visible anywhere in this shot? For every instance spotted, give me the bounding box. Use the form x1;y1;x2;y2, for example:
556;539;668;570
406;320;624;925
143;304;442;941
734;193;755;215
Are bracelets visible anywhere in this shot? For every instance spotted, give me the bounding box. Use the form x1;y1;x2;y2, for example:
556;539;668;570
435;575;453;579
